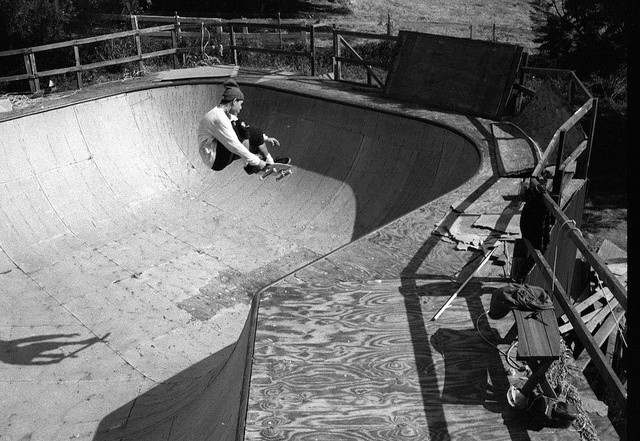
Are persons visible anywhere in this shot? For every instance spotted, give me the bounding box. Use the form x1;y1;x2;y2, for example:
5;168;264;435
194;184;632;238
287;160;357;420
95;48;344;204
198;80;291;175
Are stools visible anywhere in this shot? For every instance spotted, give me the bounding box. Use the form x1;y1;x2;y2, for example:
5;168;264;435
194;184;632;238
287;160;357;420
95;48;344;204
503;286;564;410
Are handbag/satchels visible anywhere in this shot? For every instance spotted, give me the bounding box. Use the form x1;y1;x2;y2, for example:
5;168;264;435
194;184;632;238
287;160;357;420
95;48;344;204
489;284;554;319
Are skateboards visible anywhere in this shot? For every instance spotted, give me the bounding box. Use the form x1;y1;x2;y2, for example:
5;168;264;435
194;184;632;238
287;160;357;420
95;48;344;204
259;163;292;182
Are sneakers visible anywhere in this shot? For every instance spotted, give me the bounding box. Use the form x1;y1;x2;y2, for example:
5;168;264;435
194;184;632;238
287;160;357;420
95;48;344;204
274;157;292;164
244;153;266;175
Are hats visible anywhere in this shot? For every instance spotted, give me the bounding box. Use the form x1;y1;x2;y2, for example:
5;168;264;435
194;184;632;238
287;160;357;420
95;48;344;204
223;79;244;100
